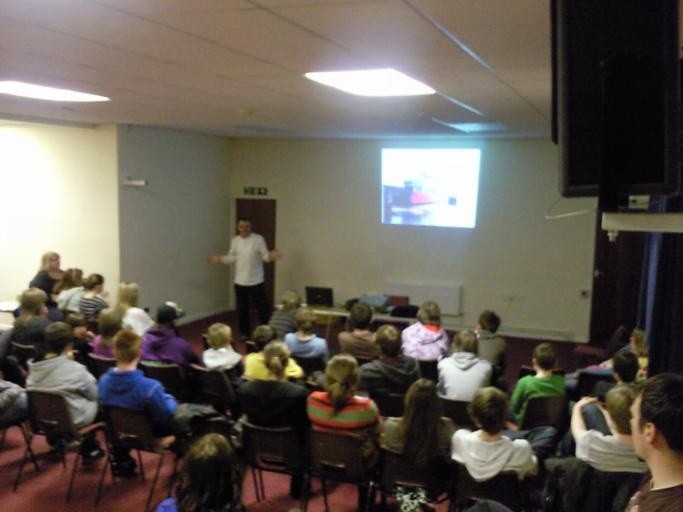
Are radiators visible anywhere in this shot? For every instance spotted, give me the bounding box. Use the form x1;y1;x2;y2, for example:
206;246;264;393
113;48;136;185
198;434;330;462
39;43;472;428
382;280;463;317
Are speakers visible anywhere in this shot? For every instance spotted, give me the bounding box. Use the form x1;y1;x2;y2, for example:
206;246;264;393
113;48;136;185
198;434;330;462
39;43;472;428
556;0;681;198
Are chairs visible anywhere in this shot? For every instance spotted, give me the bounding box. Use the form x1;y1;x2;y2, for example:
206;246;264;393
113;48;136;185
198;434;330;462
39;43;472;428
138;362;180;392
94;402;179;512
189;363;234;410
519;396;564;430
374;389;404;419
0;421;43;478
240;422;305;502
377;445;448;512
291;353;326;379
308;426;376;512
417;357;439;381
201;334;236;350
88;352;116;378
374;293;409;331
437;393;471;427
9;341;36;365
513;366;565;381
12;389;105;502
575;370;613;400
245;339;258;352
355;354;372;367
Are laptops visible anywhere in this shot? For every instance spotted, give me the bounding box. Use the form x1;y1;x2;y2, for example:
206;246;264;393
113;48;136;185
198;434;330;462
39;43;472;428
305;286;333;308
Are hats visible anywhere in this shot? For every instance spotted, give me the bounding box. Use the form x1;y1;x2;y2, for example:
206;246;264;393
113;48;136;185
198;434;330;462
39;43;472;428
156;301;185;323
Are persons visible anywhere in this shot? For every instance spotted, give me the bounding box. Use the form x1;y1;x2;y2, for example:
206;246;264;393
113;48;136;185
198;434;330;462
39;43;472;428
115;281;158;340
383;379;455;454
285;309;328;365
156;433;245;512
600;327;648;382
623;373;683;512
570;387;650;473
475;311;510;393
97;330;178;479
509;344;565;436
306;354;382;511
606;350;646;436
202;323;243;392
11;287;51;353
401;300;449;362
94;307;125;359
207;217;284;336
29;252;64;309
269;290;302;341
435;329;493;403
53;268;84;313
25;322;137;477
360;325;422;394
240;341;314;501
64;313;96;367
338;302;380;359
80;274;109;328
451;387;558;491
141;301;206;366
244;326;304;382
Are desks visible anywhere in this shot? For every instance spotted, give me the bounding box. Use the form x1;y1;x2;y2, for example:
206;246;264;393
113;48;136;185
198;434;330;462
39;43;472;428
274;302;573;343
